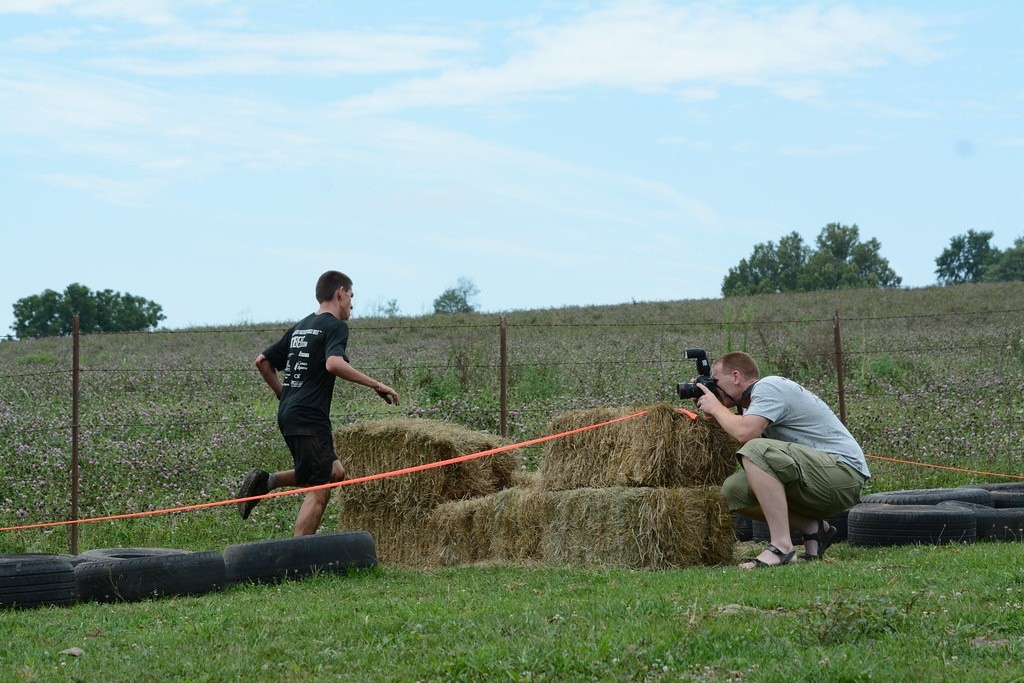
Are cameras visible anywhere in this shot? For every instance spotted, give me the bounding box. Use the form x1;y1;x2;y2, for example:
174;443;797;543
676;349;717;399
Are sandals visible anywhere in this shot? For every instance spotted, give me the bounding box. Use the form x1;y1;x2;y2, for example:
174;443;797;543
744;543;799;569
799;518;838;561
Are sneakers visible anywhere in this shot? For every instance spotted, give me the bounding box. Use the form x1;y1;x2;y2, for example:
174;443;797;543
237;467;270;520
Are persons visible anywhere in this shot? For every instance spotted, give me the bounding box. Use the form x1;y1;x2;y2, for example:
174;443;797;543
238;271;401;537
687;352;871;569
